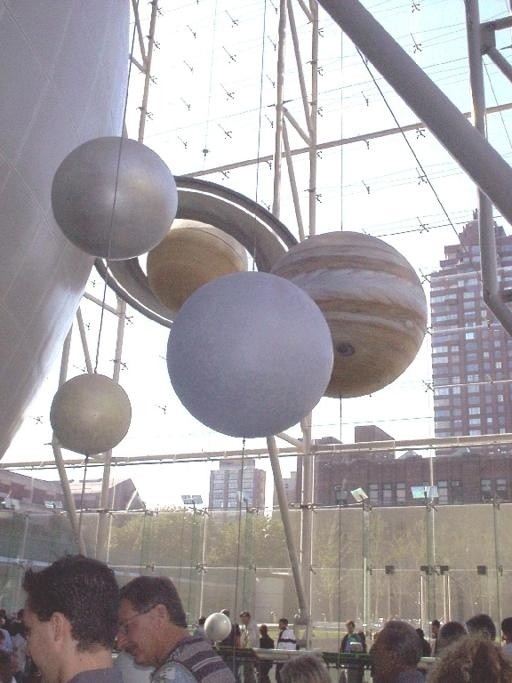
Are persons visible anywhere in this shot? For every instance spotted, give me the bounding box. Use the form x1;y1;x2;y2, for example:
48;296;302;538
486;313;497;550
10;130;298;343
112;574;238;683
329;641;330;644
1;607;42;683
21;552;125;683
189;606;512;682
